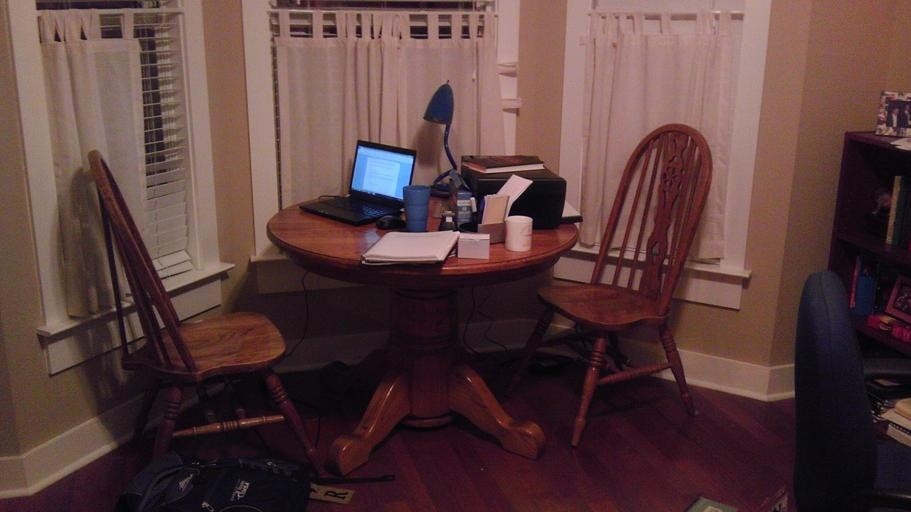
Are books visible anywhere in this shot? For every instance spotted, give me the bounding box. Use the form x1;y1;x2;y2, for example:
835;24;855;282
361;229;462;264
462;154;545;174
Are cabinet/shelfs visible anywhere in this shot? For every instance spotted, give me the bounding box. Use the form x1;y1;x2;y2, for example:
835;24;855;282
827;128;911;324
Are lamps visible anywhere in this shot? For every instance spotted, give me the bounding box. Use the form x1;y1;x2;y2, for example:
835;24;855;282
421;81;459;199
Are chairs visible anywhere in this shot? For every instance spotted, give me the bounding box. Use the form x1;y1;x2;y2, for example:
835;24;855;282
503;121;715;447
87;148;338;485
796;270;910;512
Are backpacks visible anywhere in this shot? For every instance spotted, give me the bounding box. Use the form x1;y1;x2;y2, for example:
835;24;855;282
115;459;310;512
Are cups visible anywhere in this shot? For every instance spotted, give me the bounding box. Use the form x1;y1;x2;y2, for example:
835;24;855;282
402;184;431;232
505;215;532;252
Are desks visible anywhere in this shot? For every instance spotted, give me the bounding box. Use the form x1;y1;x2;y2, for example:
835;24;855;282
265;202;581;479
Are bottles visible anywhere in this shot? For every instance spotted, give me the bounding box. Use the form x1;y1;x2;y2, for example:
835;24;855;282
455;191;471;224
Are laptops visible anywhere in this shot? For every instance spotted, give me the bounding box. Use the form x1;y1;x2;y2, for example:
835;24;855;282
298;140;416;225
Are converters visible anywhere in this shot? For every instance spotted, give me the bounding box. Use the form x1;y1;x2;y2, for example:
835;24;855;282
537;355;568;373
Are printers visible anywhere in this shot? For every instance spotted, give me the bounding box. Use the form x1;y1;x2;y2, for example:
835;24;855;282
461;155;584;230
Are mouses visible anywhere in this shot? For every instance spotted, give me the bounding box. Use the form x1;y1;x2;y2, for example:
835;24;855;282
377;215;405;229
